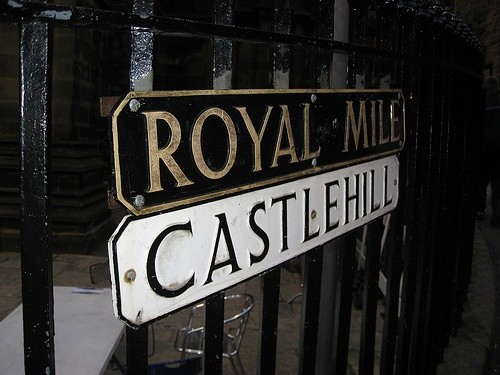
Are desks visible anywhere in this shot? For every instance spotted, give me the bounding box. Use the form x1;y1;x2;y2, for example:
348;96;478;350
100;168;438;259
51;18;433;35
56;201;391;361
0;286;126;375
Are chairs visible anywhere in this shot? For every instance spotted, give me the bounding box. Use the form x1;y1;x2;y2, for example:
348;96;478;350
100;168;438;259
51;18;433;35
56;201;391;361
146;354;204;375
174;292;256;375
